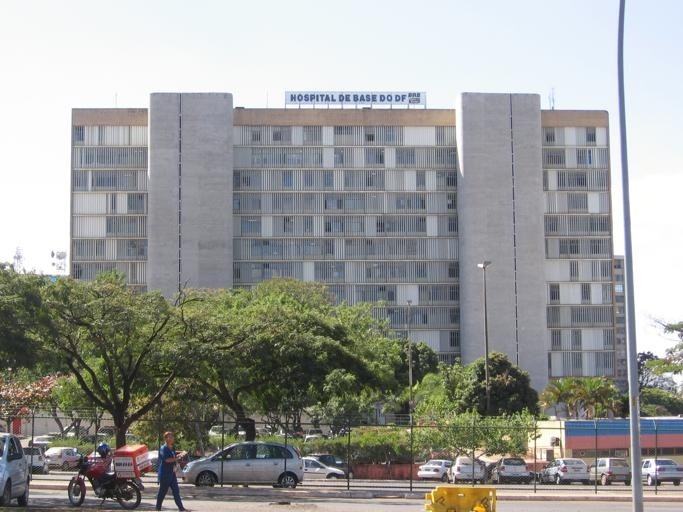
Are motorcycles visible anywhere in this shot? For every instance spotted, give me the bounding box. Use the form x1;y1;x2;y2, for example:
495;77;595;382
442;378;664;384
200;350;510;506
68;454;145;510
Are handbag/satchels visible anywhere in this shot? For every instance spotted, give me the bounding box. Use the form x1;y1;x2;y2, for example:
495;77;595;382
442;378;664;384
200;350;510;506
172;462;185;478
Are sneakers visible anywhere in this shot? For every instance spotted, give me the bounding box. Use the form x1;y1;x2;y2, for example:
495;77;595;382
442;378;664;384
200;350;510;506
177;508;192;512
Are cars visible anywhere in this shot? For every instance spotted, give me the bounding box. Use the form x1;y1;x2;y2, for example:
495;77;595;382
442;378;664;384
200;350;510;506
43;447;82;471
209;424;357;444
641;457;682;487
147;450;160;471
175;451;190;468
31;424;140;447
446;457;487;485
311;453;353;480
0;433;31;507
537;458;589;485
416;460;452;482
87;452;103;466
300;456;345;482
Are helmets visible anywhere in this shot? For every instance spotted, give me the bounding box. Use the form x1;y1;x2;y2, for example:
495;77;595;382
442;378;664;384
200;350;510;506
96;443;110;458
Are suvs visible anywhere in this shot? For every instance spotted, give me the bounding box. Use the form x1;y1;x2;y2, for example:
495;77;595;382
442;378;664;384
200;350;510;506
588;457;631;486
490;457;532;485
22;446;48;475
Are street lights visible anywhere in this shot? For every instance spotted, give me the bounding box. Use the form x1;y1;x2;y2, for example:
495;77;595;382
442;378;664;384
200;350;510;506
404;297;416;427
477;258;492;419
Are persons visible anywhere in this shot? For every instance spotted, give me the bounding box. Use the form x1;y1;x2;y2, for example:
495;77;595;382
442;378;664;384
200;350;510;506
155;432;187;512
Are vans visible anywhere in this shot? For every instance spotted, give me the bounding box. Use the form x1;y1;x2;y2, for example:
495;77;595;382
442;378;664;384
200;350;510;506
181;441;304;491
